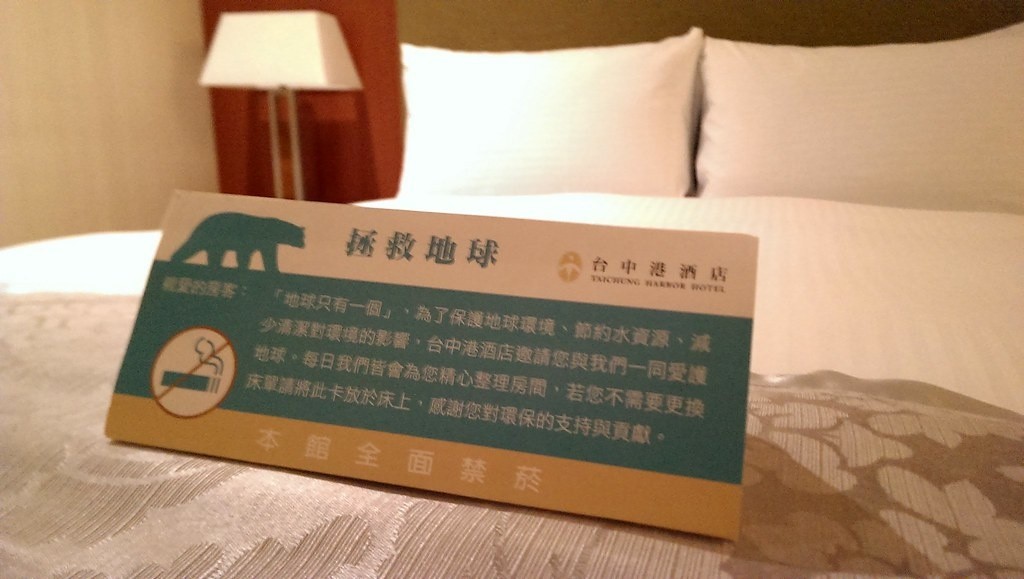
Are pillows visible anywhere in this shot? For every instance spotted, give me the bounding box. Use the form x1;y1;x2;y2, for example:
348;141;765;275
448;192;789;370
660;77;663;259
395;24;703;196
695;23;1024;216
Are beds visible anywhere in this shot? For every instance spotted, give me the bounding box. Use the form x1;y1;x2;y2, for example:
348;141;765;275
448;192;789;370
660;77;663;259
0;191;1024;579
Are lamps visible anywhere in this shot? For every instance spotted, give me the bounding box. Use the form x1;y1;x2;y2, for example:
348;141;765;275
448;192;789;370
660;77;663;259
198;12;364;200
